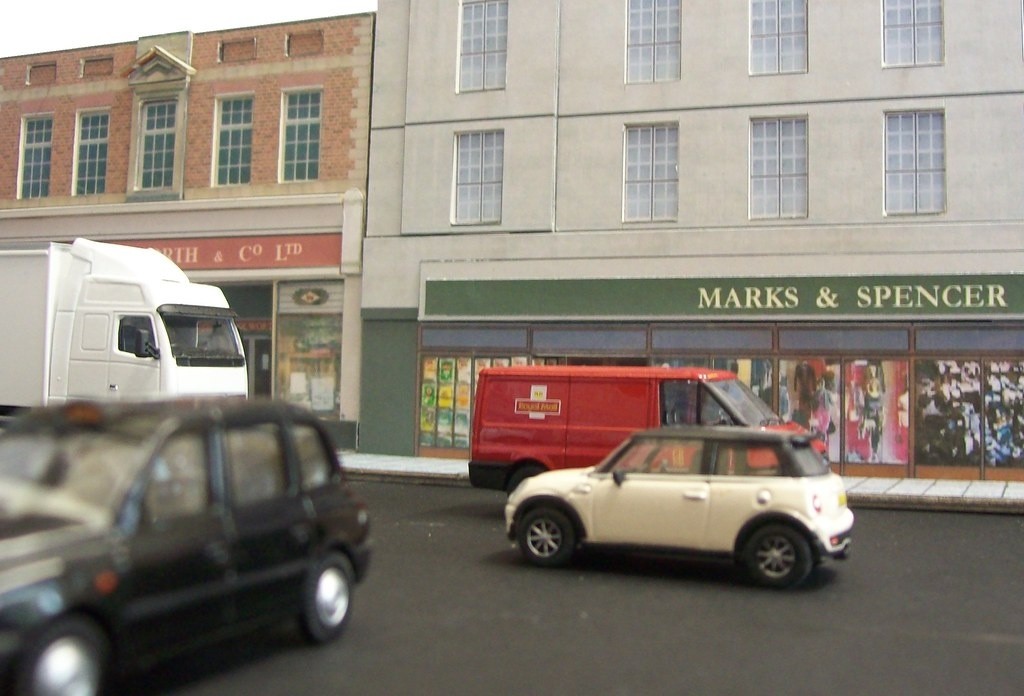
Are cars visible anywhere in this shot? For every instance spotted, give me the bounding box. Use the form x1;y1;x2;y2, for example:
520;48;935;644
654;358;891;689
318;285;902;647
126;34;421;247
506;428;855;593
0;397;371;696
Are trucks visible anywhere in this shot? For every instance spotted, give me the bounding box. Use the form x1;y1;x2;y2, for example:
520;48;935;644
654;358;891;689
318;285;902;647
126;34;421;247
0;237;249;418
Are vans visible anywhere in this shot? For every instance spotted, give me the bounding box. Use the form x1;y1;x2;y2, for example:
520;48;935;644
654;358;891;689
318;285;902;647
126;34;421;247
468;367;829;497
